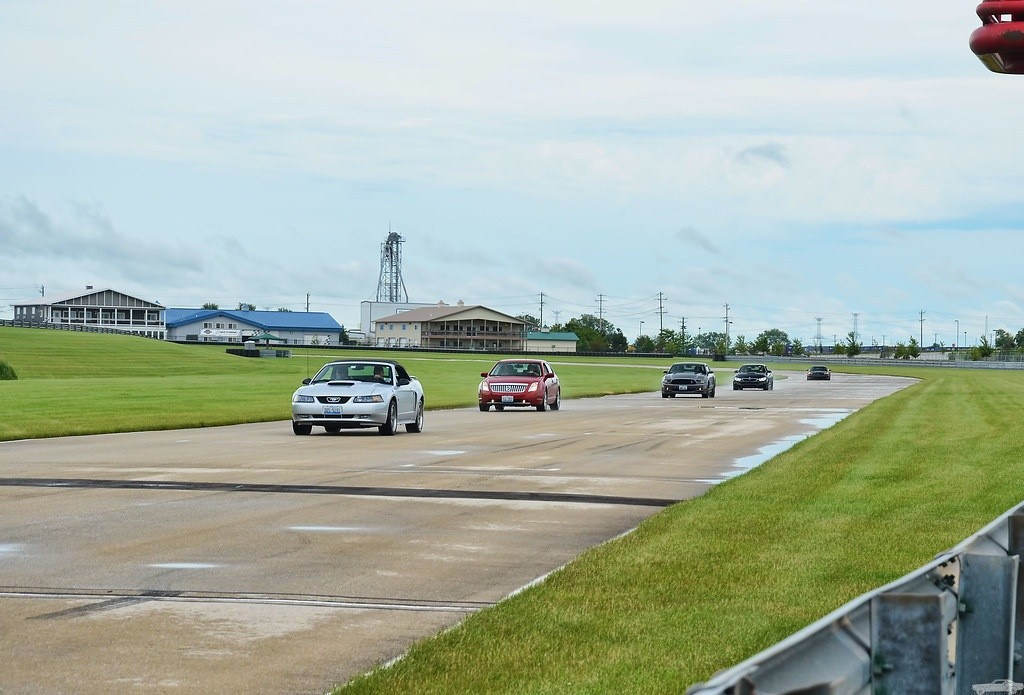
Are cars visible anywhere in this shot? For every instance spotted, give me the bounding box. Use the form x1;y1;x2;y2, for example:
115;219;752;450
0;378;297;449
732;363;774;391
477;358;561;412
290;358;424;436
661;361;716;398
806;364;832;380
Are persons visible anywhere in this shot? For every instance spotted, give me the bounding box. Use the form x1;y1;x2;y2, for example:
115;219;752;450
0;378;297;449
526;365;539;376
373;366;391;383
693;367;704;374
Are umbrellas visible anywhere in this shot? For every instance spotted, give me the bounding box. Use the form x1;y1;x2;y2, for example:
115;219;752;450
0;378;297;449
248;333;287;350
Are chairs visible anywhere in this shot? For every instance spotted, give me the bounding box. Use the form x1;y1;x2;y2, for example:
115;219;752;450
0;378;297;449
336;367;347;378
532;366;538;373
506;365;514;373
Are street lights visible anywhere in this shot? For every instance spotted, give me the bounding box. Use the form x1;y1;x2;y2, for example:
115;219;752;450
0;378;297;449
639;320;644;336
